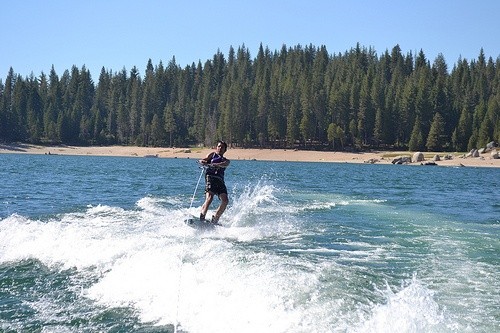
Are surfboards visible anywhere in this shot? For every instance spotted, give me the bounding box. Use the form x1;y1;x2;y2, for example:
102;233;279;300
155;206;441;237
184;218;211;228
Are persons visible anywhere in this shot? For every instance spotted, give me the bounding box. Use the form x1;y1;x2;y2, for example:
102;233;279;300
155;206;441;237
198;140;230;225
